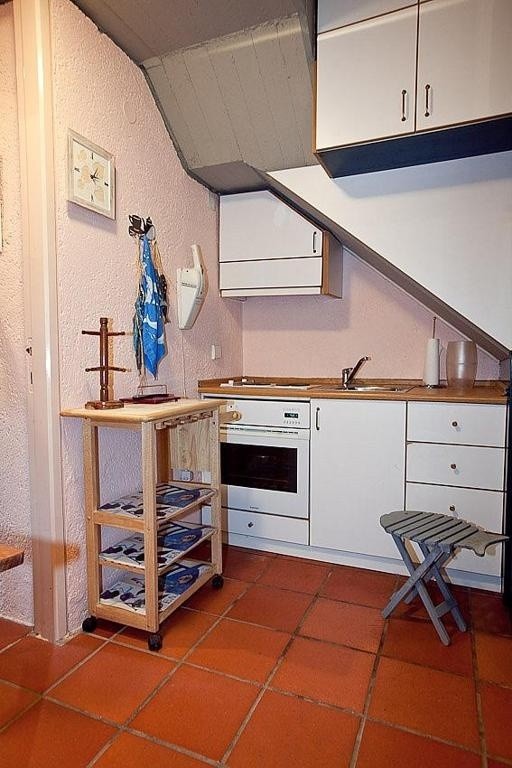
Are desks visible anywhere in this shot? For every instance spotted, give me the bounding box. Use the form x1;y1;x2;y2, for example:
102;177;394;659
0;544;24;573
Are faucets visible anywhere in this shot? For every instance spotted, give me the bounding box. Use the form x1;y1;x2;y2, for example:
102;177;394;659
341;357;371;385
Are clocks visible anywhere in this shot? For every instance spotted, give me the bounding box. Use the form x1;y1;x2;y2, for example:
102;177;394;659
68;128;117;221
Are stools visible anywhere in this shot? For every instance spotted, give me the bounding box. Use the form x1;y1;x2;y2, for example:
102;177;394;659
380;510;507;647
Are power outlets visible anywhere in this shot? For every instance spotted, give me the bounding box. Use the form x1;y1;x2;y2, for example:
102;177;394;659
212;344;223;360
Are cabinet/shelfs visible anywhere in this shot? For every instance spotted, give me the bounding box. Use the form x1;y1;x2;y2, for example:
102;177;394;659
404;400;509;596
218;189;343;301
59;398;228;651
308;398;406;577
314;1;511;180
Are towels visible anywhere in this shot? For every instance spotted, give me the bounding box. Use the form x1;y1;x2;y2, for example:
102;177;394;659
135;234;168;380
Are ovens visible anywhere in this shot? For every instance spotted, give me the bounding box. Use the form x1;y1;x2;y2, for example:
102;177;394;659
201;394;309;547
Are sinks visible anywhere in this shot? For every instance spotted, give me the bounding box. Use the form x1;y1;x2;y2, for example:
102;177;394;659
308;382;417;394
